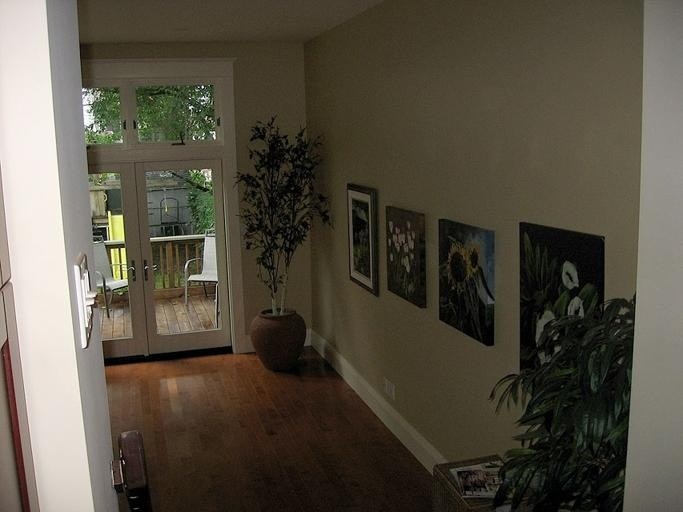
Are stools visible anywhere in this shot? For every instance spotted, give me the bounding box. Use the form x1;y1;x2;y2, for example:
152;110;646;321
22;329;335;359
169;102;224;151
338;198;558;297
432;453;537;511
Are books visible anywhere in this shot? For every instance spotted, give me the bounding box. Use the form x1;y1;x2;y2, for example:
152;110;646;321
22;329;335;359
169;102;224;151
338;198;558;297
449;460;518;499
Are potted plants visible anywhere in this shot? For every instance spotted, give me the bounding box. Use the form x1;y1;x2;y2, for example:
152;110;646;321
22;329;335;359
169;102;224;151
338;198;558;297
227;110;335;372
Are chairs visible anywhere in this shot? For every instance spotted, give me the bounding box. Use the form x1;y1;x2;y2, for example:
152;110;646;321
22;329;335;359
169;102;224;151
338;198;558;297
181;227;219;306
93;234;128;319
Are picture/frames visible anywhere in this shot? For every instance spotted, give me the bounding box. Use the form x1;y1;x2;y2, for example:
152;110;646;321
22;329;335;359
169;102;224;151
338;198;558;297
347;184;379;299
